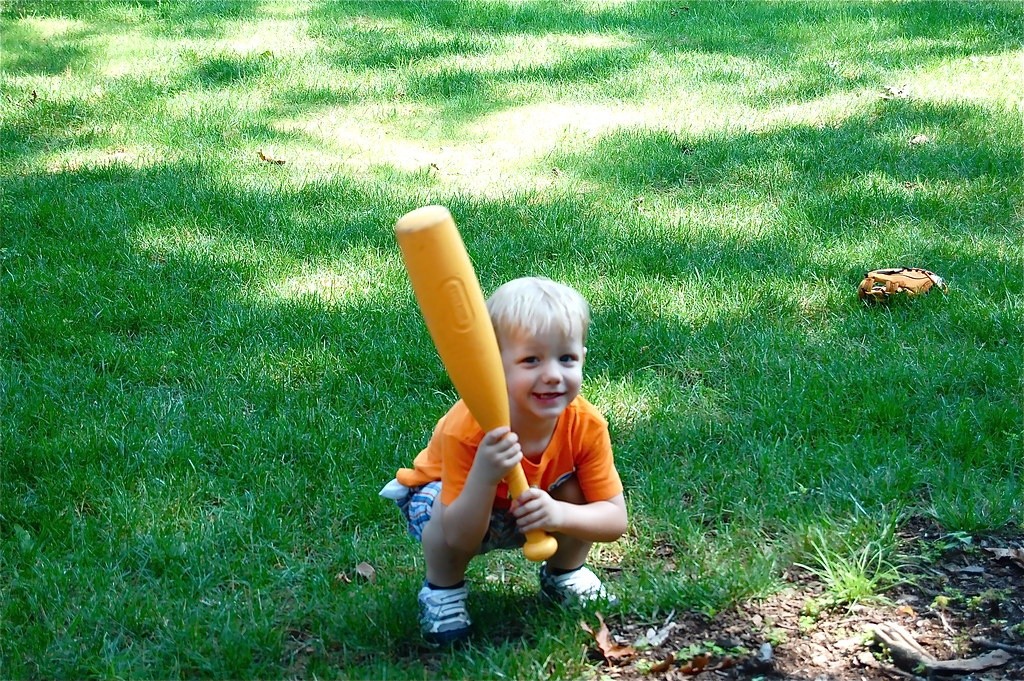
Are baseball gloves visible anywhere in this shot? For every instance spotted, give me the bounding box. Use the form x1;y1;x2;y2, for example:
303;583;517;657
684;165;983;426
856;268;940;311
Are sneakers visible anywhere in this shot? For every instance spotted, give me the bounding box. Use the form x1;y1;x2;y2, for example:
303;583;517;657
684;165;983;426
540;557;617;611
416;579;474;648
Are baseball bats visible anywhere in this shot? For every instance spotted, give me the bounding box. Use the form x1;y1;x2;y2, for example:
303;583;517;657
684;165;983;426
396;205;560;561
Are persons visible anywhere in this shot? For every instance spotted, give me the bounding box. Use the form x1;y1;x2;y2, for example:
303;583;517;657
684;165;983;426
377;276;628;647
